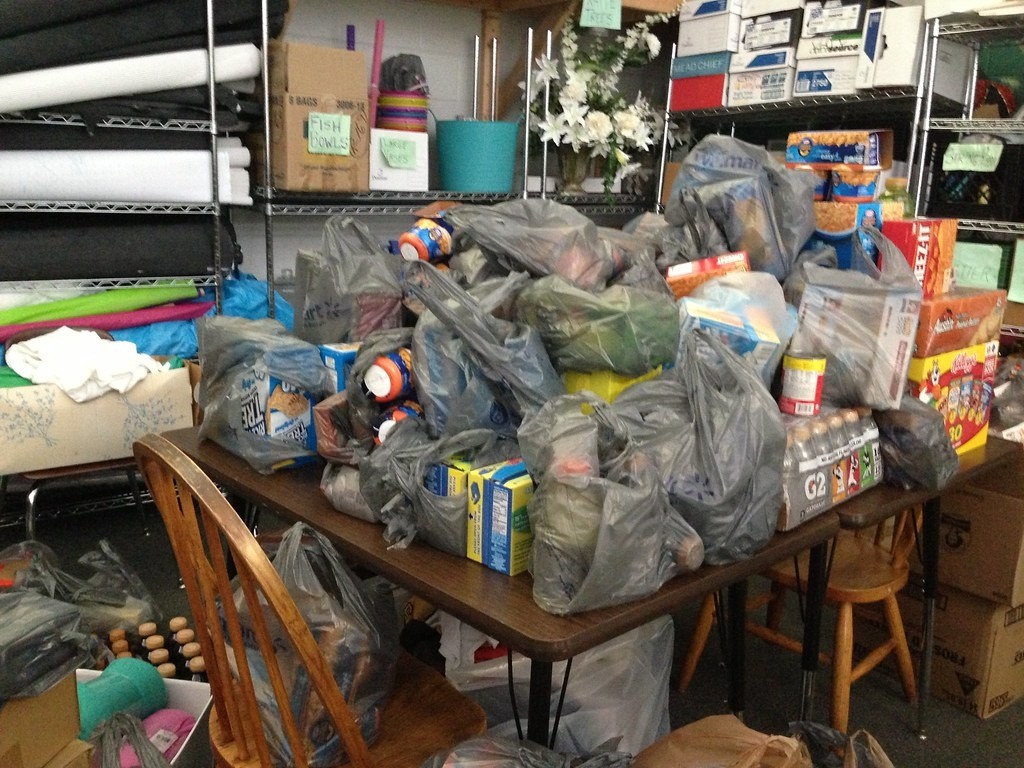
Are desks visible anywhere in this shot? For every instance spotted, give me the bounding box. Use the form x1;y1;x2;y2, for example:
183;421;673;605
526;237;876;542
834;410;1024;745
151;426;840;748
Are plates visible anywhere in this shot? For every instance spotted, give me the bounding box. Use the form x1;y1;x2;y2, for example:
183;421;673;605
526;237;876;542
376;90;428;132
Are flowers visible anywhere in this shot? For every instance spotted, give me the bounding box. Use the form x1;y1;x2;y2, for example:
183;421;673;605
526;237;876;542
515;3;690;153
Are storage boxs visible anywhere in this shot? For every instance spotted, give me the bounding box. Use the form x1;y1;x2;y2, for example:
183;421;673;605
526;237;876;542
0;0;1024;768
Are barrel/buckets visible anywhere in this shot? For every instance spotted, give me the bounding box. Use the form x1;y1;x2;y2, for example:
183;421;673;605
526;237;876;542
429;109;524;191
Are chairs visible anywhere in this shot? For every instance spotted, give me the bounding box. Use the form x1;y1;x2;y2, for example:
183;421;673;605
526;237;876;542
132;435;486;767
669;494;928;759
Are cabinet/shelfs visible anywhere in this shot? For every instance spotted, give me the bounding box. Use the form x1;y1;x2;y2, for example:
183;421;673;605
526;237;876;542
0;107;235;527
908;14;1024;245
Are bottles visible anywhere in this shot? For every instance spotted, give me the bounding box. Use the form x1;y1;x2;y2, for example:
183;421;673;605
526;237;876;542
777;407;882;531
95;617;208;683
779;352;826;416
877;177;915;218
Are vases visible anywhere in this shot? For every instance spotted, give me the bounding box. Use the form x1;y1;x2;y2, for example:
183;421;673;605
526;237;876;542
555;140;589;195
